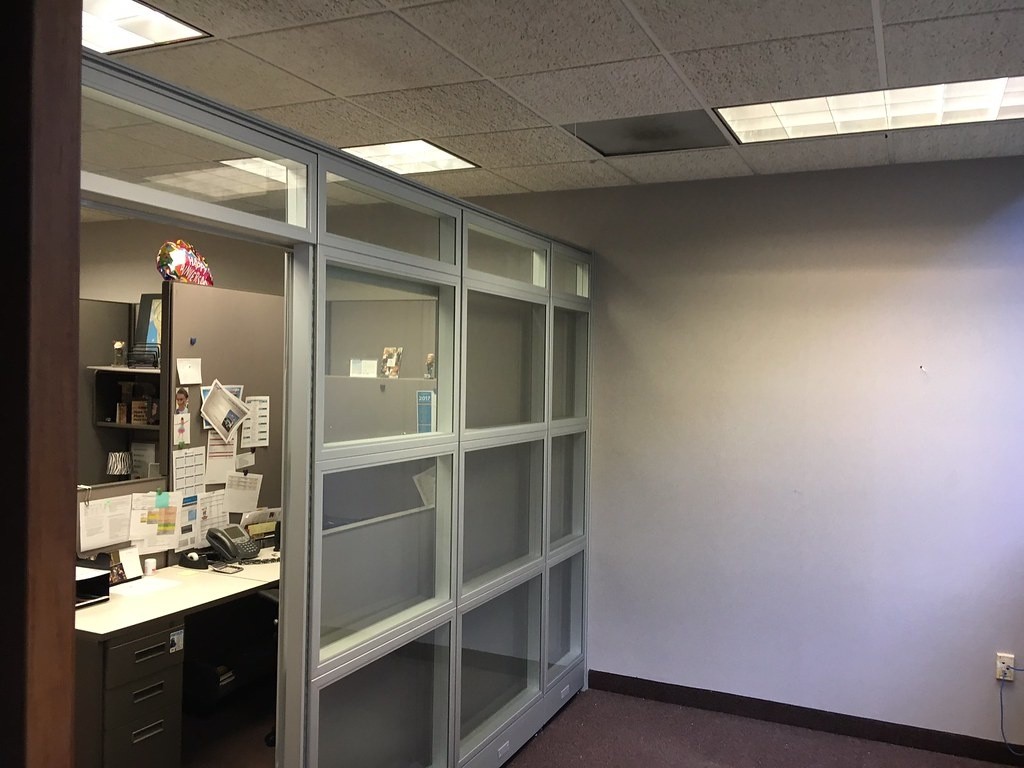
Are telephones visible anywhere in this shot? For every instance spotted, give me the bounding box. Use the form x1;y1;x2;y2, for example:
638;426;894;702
205;523;261;564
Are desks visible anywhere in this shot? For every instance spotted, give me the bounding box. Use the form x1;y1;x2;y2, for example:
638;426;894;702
75;546;278;768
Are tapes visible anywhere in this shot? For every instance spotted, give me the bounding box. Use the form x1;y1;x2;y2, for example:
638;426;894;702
188;552;199;561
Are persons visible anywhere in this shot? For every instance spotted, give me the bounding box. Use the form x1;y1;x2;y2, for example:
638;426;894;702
151;402;159;419
176;388;189;414
112;566;124;581
427;353;434;374
175;417;189;439
